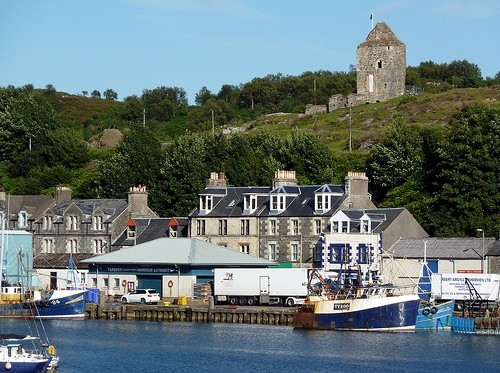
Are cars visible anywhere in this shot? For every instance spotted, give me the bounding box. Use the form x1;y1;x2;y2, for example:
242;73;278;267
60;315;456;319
120;288;160;305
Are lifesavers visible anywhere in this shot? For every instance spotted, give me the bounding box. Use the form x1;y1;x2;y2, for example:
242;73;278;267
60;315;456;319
326;292;335;300
122;280;126;286
48;345;56;355
168;280;173;287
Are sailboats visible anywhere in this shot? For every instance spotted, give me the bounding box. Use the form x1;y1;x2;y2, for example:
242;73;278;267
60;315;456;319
0;210;61;373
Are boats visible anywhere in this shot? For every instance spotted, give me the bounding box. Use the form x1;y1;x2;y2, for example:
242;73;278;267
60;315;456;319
374;237;455;333
0;243;88;322
443;277;500;335
282;241;420;334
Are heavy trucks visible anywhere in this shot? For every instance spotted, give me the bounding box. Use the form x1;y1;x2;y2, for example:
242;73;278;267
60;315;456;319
428;273;500;312
211;268;344;309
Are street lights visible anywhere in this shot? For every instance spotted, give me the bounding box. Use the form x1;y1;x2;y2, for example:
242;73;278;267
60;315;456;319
477;228;486;273
169;263;181;297
